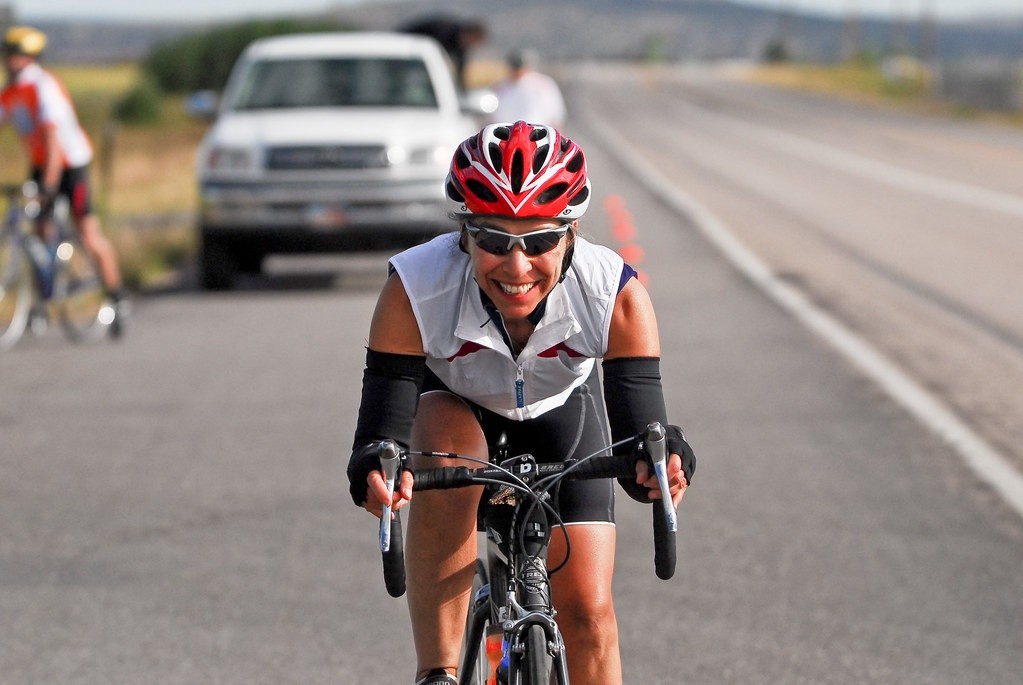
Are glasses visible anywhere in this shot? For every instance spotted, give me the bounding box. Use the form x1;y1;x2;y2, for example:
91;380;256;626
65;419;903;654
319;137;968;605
464;223;569;256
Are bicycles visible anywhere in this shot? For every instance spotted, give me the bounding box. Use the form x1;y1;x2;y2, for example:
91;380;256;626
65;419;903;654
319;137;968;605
0;184;112;352
378;421;679;685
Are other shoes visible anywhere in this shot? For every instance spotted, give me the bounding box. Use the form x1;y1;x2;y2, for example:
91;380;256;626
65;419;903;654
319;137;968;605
109;290;129;340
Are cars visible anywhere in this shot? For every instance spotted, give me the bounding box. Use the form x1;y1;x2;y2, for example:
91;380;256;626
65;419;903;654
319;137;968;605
185;29;499;289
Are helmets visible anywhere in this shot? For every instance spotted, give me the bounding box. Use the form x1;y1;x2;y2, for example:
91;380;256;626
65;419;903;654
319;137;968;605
445;120;590;219
0;26;47;56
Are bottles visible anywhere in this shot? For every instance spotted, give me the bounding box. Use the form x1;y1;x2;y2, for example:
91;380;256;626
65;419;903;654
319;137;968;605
496;628;519;685
485;622;503;685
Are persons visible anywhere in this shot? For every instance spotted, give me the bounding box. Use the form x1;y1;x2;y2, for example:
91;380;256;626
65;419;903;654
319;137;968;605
485;46;569;131
393;11;485;97
345;120;696;685
0;25;133;341
